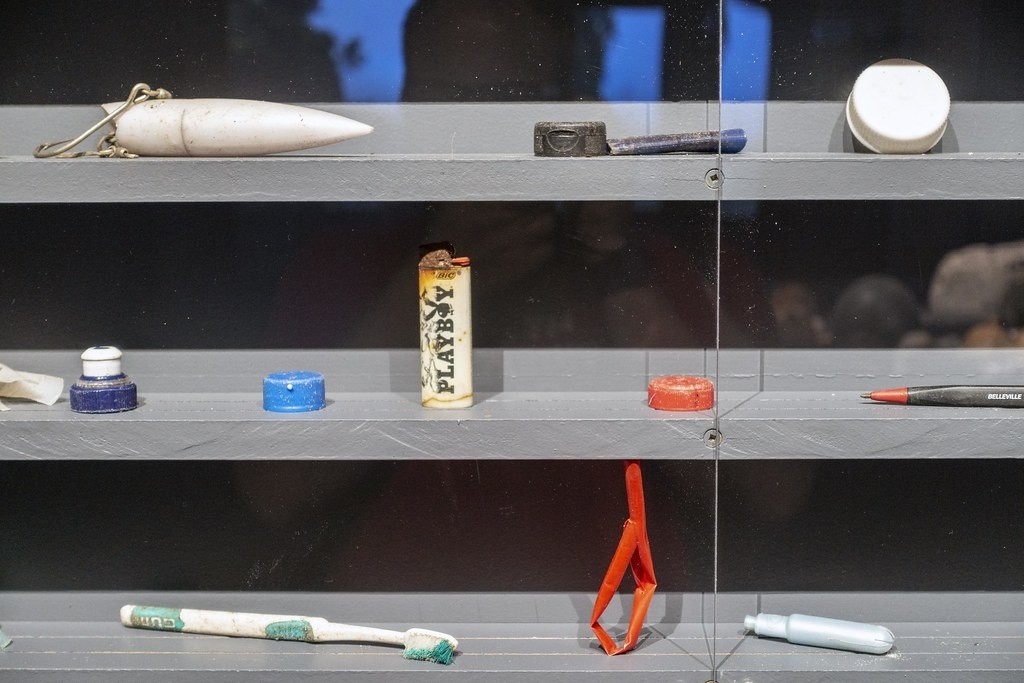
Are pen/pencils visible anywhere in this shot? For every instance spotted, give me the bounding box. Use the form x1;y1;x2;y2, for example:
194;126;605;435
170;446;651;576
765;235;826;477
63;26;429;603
860;384;1024;409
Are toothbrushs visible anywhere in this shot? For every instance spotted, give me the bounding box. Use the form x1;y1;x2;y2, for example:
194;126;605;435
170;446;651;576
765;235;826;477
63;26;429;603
120;604;458;666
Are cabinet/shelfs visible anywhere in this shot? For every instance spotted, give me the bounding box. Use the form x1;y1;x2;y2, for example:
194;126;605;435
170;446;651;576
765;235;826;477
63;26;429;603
0;102;1024;683
